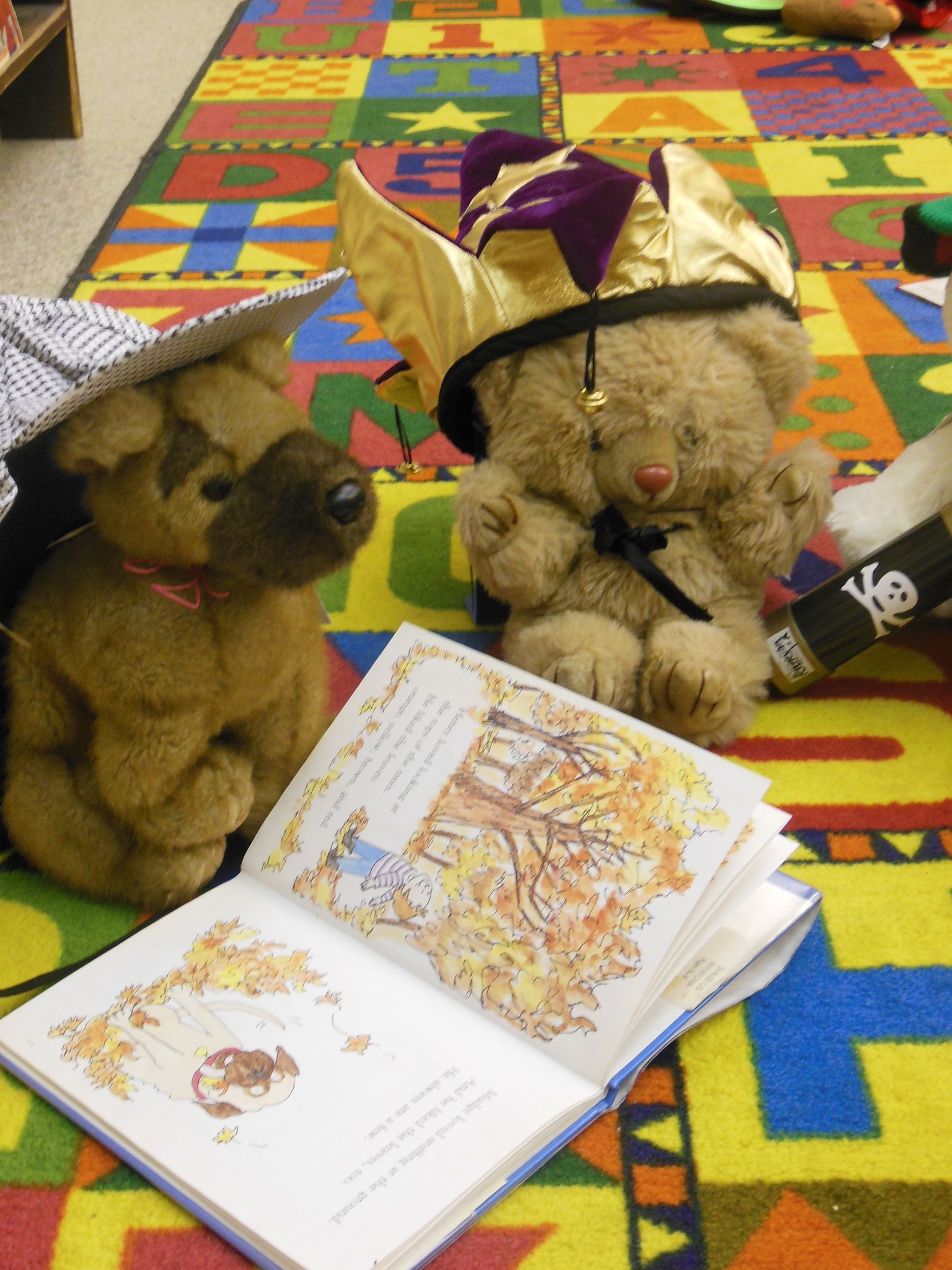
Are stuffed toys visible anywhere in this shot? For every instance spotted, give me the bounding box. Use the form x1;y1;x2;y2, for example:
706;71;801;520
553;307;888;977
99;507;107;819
328;124;839;752
1;271;380;911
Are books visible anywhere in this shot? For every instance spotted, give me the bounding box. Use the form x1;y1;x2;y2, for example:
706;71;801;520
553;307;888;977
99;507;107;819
1;623;827;1269
